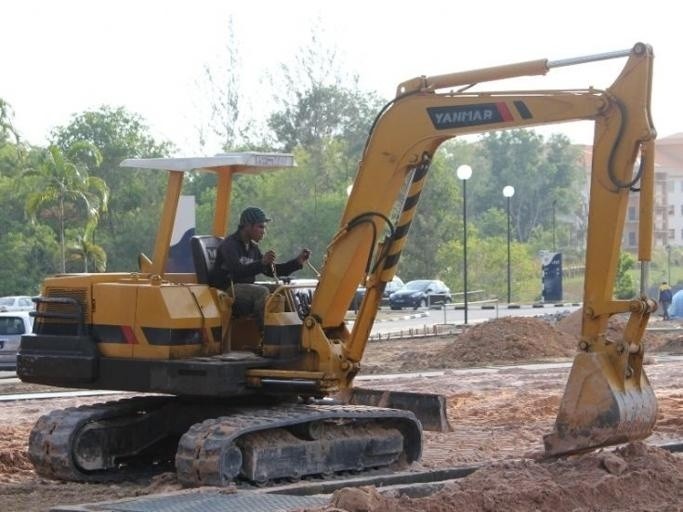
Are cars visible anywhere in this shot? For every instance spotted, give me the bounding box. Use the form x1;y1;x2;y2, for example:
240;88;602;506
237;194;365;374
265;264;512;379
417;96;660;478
0;296;36;312
348;285;365;309
389;279;453;309
284;279;319;303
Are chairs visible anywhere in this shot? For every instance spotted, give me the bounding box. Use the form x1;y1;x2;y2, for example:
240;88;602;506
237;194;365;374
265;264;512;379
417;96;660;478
190;233;225;284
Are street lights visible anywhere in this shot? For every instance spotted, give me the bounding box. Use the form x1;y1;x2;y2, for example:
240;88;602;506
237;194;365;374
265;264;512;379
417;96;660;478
501;186;516;304
455;164;473;325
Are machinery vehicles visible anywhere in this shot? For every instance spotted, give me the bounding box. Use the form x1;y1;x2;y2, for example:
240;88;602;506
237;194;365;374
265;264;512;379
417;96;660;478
15;40;659;491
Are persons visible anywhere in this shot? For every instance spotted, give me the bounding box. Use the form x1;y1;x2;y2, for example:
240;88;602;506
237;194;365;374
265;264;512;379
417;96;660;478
659;282;672;321
210;207;311;354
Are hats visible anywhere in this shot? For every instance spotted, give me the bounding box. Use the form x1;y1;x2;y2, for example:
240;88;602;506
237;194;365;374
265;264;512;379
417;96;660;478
240;208;272;225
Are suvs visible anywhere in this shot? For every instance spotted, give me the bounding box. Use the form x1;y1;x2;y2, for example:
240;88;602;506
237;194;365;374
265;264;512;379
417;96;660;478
0;312;36;373
380;273;404;306
